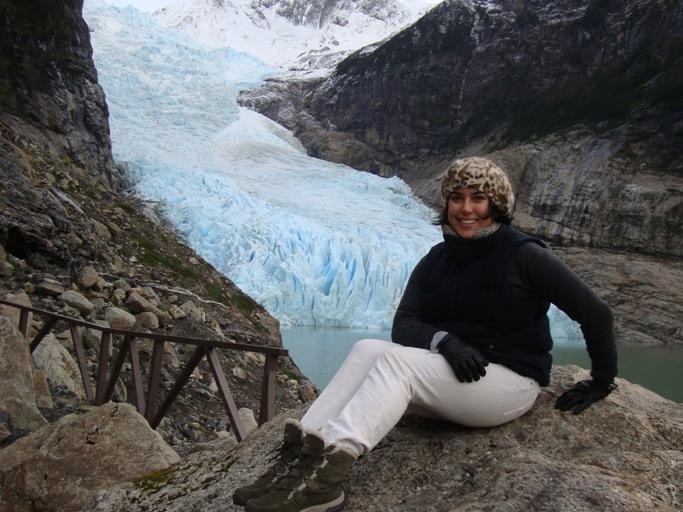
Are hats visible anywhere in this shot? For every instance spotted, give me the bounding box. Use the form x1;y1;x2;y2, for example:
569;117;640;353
440;157;516;219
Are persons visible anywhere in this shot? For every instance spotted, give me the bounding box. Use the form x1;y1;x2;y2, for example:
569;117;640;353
231;156;619;511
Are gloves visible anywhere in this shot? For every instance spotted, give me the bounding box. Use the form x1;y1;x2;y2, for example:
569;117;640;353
436;333;489;384
553;377;617;415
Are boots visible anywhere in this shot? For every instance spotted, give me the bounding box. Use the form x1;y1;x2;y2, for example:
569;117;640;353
231;416;360;512
